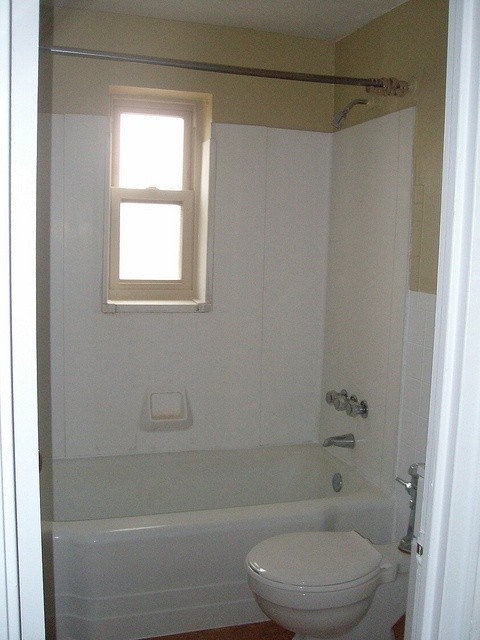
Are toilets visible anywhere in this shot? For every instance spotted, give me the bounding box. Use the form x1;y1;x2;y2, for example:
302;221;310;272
243;463;423;640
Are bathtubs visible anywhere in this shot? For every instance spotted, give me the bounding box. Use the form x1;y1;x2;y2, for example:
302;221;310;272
40;443;397;638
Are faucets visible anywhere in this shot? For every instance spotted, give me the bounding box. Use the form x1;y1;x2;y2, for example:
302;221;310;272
323;435;353;450
